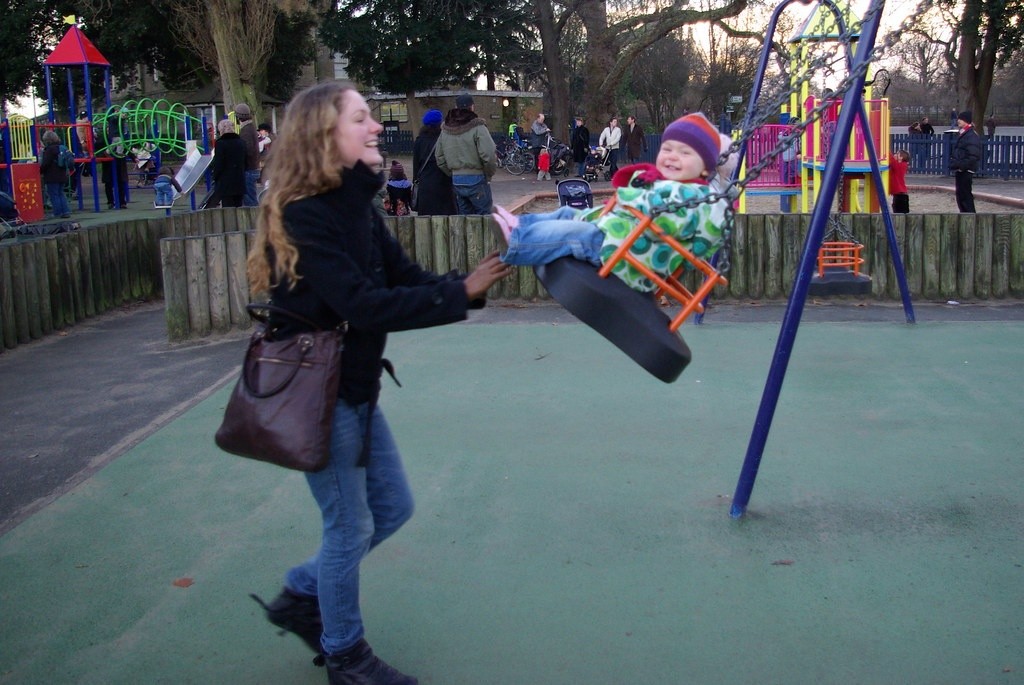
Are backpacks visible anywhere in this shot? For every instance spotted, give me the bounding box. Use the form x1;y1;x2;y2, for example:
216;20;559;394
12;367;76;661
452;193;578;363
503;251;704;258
50;143;75;169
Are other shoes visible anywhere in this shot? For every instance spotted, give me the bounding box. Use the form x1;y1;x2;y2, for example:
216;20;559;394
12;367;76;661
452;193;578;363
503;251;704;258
490;203;518;251
61;213;70;218
109;203;114;209
122;205;127;209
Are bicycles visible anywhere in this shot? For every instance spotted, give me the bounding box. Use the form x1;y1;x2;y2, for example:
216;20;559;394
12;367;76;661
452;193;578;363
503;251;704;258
492;133;538;176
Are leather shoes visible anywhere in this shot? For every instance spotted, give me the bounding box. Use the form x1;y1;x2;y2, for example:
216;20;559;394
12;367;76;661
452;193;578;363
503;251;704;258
312;639;419;685
249;587;326;653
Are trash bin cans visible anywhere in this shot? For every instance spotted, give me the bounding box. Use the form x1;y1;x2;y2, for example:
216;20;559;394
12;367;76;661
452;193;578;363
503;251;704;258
944;129;959;155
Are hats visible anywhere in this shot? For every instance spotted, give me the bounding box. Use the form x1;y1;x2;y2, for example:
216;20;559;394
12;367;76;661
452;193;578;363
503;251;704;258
959;111;973;125
234;103;251;115
390;160;405;176
455;93;476;109
661;113;721;175
423;111;443;126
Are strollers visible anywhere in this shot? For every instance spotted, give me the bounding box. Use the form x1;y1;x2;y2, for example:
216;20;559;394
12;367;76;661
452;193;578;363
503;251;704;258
538;133;575;178
580;144;612;183
556;179;594;209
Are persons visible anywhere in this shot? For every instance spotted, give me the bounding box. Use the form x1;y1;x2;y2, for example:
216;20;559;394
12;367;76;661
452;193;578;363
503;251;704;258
921;117;934;136
77;110;96;154
599;117;622;179
436;93;496;214
152;166;186;206
889;149;910;212
536;149;552;181
986;115;996;135
909;123;921;153
134;142;156;189
495;112;736;285
570;118;590;176
414;109;459;215
949;110;981;212
257;124;272;155
531;114;550;177
99;126;128;210
388;160;412;216
509;119;517;139
779;117;799;183
204;120;247;209
104;107;127;157
586;145;601;167
951;108;957;128
235;104;260;206
244;81;518;684
39;131;74;217
623;115;648;164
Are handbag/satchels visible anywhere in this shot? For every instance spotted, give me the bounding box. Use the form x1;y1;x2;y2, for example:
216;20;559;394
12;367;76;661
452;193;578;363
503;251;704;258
215;302;345;473
409;179;419;211
